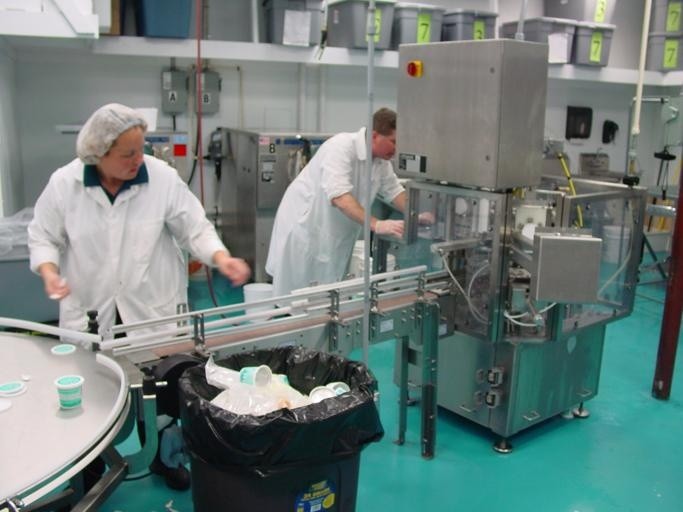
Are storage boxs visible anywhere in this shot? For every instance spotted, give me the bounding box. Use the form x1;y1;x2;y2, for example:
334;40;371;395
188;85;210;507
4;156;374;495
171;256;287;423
391;2;446;49
644;33;683;73
442;9;497;44
326;0;396;51
120;1;193;41
573;19;618;69
261;1;326;47
649;0;683;34
542;1;614;23
497;14;577;66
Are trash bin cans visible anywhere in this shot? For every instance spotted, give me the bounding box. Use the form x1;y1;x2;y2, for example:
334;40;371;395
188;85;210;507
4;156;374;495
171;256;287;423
177;345;384;511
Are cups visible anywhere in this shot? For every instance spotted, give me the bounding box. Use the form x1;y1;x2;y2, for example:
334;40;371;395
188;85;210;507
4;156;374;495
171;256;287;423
54;376;84;409
238;365;272;387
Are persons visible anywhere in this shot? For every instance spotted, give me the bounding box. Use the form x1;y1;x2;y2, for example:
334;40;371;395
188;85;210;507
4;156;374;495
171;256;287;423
264;108;435;309
24;102;253;495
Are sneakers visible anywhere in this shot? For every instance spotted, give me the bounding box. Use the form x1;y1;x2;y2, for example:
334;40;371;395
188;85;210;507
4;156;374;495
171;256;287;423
150;460;189;490
